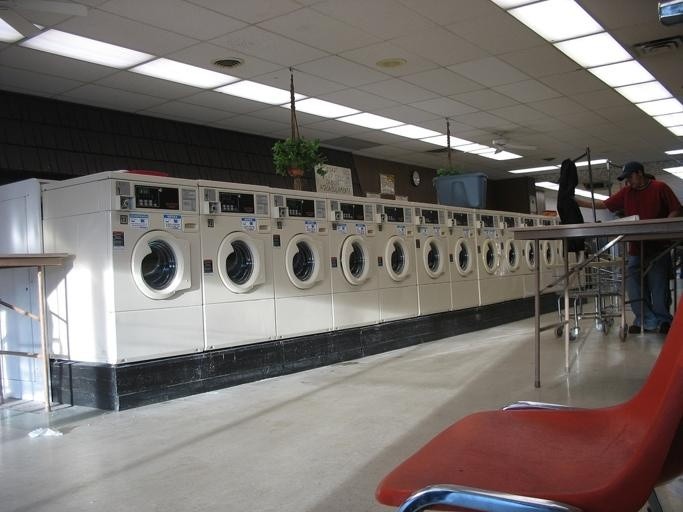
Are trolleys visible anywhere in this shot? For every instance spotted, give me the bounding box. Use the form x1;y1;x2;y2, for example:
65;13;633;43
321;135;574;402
546;254;628;341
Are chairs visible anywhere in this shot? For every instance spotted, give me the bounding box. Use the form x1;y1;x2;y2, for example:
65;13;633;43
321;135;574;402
373;279;682;512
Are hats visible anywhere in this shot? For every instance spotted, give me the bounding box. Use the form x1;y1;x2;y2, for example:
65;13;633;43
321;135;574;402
616;161;644;182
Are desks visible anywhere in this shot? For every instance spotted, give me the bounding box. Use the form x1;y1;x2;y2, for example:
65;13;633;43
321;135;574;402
0;250;71;414
512;214;682;388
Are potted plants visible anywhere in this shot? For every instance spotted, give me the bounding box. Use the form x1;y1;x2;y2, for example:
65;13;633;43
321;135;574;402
270;132;329;180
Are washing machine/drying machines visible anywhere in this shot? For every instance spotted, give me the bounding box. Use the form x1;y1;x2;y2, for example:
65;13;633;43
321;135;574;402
38;178;587;367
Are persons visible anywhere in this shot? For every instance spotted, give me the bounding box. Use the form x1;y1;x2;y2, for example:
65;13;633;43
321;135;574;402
575;161;682;334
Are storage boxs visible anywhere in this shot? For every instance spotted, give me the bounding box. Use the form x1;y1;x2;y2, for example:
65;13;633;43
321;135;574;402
432;172;489;209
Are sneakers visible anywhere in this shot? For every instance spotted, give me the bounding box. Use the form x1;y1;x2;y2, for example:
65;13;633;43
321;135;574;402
628;322;671;335
